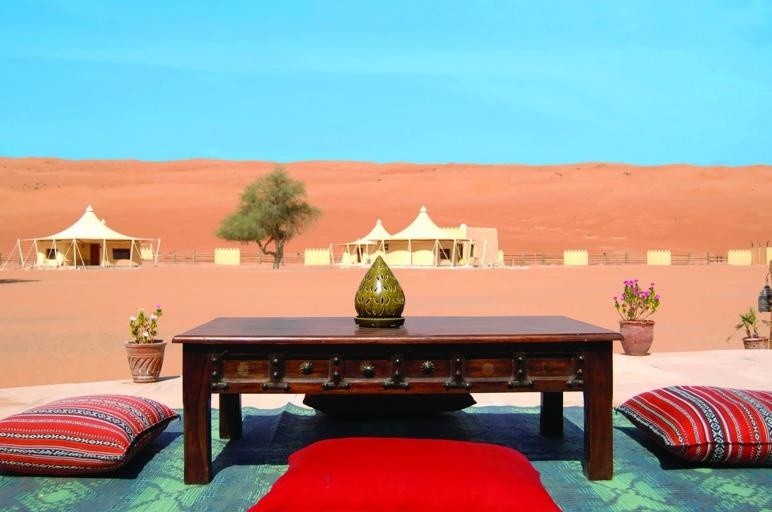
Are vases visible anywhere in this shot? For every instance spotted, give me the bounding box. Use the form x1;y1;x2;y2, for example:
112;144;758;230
620;321;654;356
126;340;166;383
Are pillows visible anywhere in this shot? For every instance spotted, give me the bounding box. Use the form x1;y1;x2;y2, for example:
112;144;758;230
615;385;772;466
244;436;560;511
0;395;181;477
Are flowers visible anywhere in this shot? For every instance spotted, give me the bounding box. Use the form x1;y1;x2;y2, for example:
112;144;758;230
612;279;662;321
129;304;163;344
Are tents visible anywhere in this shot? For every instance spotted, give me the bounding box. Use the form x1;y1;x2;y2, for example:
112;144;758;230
5;205;157;269
384;205;473;268
329;218;392;267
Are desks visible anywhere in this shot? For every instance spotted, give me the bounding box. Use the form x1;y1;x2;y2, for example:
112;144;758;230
171;318;625;485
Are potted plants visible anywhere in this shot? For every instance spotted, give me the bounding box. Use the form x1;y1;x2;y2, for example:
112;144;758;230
736;306;769;349
